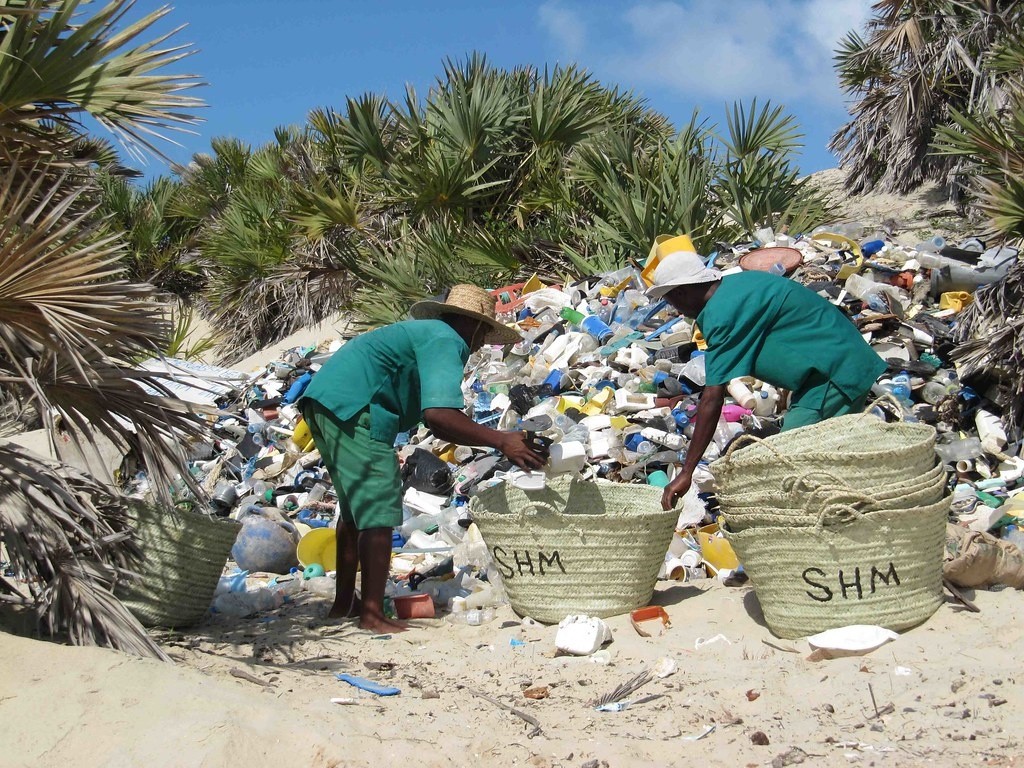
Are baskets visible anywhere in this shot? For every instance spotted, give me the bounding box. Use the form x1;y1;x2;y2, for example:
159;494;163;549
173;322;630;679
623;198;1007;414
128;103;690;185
707;394;955;641
467;460;684;624
84;498;244;628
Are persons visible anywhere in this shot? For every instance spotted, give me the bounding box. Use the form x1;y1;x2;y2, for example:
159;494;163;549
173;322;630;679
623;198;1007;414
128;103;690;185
645;251;888;511
297;284;548;633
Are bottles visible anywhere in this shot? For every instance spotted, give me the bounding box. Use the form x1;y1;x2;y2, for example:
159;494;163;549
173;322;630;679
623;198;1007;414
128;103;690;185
134;217;1024;627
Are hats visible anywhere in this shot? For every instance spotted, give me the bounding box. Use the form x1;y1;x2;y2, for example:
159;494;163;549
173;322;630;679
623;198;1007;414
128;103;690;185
644;251;722;299
410;284;526;345
